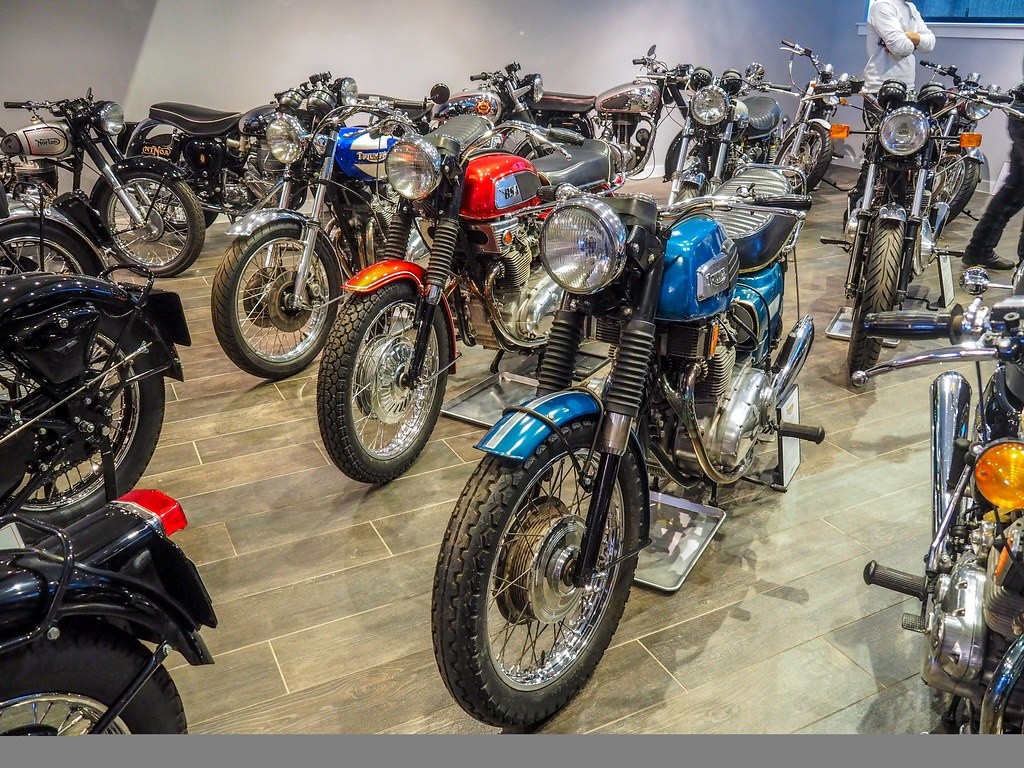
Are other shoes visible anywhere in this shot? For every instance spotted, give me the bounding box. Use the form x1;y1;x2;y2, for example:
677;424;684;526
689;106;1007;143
961;243;1015;270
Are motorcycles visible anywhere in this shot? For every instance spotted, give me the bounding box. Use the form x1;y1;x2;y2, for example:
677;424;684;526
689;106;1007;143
853;266;1024;734
0;86;205;278
0;181;112;280
779;40;871;195
1;264;191;545
317;112;626;483
525;45;695;183
636;45;800;206
819;59;1024;387
117;70;357;235
431;163;826;728
0;488;218;736
358;61;567;162
211;84;403;378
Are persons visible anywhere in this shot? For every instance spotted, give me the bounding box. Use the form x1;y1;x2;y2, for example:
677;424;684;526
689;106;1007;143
841;0;936;233
962;54;1024;285
